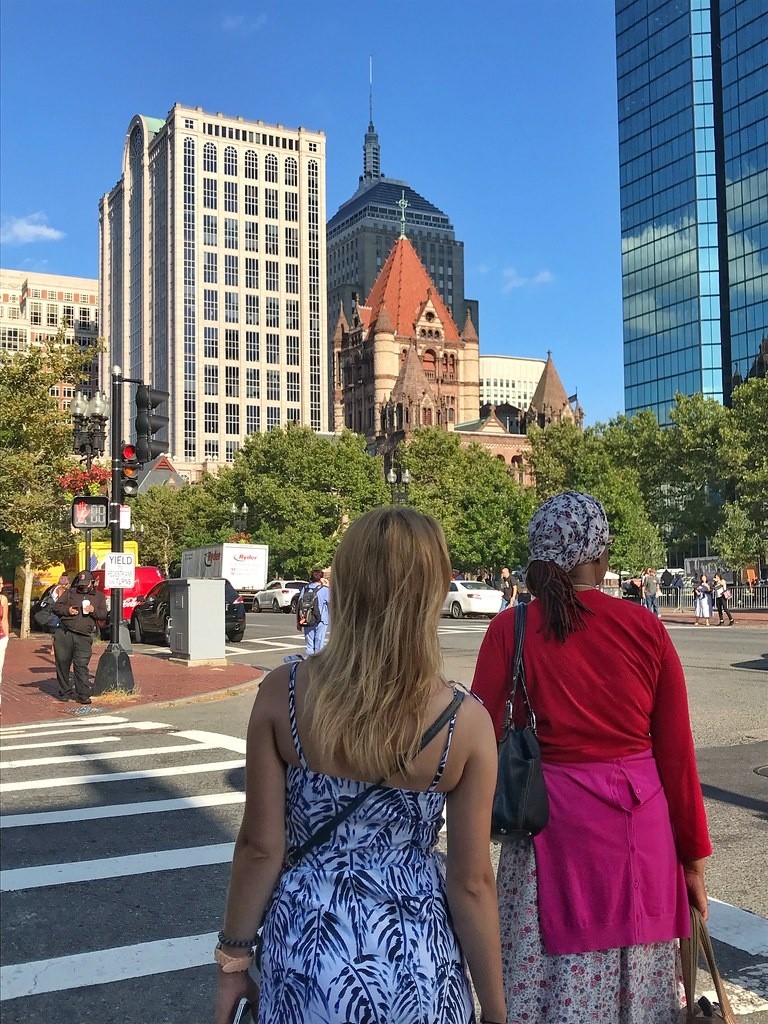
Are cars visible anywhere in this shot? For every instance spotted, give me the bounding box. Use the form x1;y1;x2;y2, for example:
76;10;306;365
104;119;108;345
439;580;504;619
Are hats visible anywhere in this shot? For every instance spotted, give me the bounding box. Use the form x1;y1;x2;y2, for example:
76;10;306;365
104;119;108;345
77;571;93;585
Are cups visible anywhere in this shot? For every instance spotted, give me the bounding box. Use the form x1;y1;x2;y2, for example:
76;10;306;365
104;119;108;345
82;600;90;615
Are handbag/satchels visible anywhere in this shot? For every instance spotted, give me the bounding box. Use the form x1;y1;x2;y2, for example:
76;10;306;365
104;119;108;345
34;610;53;628
490;726;550;837
687;996;735;1024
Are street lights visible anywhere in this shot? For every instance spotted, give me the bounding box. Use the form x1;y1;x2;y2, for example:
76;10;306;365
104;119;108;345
70;390;110;570
388;468;410;506
230;503;249;539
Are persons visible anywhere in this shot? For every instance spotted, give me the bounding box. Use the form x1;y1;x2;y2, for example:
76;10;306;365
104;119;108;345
456;572;499;589
212;505;508;1024
50;576;70;602
499;565;526;612
471;493;713;1024
52;570;107;704
625;567;734;627
297;569;329;657
0;576;9;715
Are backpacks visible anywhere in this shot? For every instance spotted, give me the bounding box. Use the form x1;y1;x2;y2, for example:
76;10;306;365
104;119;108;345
297;585;329;627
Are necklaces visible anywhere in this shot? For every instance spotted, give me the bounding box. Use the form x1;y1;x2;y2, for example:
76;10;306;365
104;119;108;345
573;582;594;589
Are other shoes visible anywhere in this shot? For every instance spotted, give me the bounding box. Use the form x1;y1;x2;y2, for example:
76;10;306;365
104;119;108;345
705;621;709;626
717;621;724;626
76;694;91;704
694;622;699;625
59;693;72;702
729;619;734;626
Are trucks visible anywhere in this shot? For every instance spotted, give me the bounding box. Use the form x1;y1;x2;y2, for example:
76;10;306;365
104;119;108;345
168;543;269;611
13;540;138;630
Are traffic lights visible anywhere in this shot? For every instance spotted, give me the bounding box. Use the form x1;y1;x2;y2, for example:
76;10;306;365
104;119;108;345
121;440;138;497
73;496;109;528
136;384;169;463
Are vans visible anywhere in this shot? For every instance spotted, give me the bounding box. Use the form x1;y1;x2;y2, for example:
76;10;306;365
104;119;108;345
91;567;163;638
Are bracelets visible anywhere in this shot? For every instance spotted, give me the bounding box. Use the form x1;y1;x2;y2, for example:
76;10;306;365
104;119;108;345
217;931;261;947
214;942;255;972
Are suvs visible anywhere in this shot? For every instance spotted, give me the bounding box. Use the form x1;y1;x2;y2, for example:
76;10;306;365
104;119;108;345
251;579;309;613
131;576;246;649
